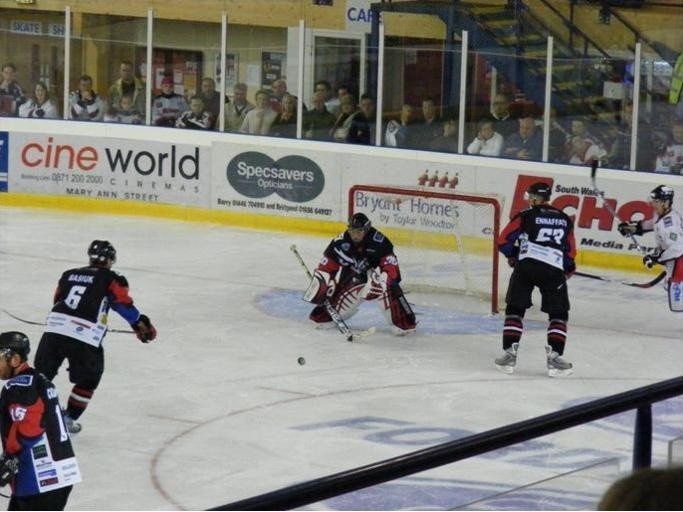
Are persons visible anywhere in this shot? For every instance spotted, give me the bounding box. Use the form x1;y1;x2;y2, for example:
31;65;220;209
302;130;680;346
618;185;683;312
34;240;156;433
598;465;683;510
534;99;683;174
69;60;146;125
384;96;472;155
151;76;230;132
466;94;543;162
215;79;308;139
0;331;83;511
302;81;388;147
303;213;417;336
494;182;576;369
2;62;60;120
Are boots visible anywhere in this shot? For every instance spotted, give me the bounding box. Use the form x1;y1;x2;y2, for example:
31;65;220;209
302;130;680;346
62;411;82;434
545;344;573;370
494;342;519;368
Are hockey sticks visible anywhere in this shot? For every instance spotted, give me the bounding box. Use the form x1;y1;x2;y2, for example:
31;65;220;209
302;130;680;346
574;271;668;288
2;308;137;334
590;160;655;269
290;243;377;342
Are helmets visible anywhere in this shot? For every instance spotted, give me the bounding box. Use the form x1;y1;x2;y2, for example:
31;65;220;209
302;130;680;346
522;181;552;202
0;330;30;350
86;240;116;266
649;184;674;199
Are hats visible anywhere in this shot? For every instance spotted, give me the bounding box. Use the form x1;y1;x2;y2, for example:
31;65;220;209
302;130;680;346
160;75;173;85
347;212;371;233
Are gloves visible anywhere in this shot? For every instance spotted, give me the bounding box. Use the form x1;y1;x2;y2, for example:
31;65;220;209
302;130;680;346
0;455;21;487
642;248;666;270
618;219;644;239
132;314;157;343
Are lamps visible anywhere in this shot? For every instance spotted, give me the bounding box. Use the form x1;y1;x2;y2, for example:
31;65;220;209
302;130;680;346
598;3;611;25
